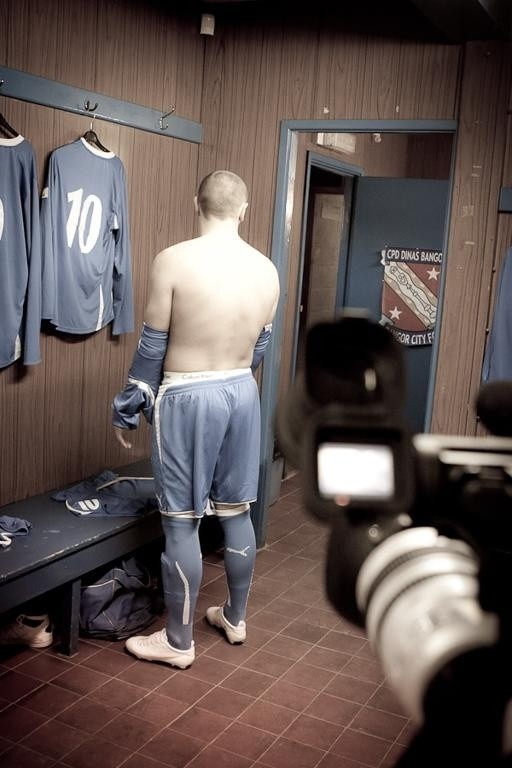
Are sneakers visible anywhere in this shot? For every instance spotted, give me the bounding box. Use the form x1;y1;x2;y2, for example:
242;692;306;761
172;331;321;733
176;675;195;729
6;615;53;648
126;628;195;669
207;606;247;644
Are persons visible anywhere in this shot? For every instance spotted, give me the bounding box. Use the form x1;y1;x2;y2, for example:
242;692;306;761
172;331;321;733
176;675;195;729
111;170;281;671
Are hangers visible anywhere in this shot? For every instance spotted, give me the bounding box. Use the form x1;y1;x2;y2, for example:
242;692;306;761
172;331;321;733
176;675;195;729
1;114;18;141
85;108;110;153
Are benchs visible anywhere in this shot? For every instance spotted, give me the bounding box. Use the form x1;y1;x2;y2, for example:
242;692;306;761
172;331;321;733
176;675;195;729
0;459;163;656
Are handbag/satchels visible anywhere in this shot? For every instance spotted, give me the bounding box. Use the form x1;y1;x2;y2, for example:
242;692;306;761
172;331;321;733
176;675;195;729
81;553;165;640
